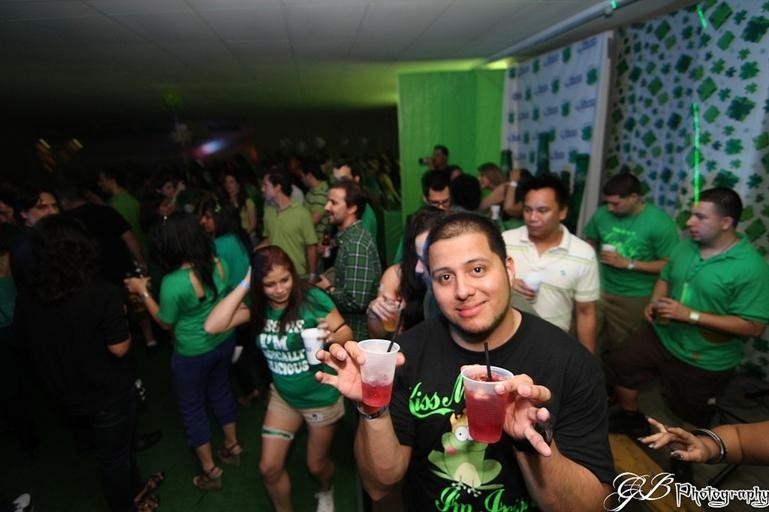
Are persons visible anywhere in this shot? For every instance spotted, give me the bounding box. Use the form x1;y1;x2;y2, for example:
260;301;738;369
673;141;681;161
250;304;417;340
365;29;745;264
1;133;768;512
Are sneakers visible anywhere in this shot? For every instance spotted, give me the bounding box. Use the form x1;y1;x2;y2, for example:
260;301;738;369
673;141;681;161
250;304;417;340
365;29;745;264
13;494;34;511
314;486;335;512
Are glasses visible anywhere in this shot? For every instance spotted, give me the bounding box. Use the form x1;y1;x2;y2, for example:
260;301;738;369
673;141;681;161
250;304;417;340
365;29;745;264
431;198;451;208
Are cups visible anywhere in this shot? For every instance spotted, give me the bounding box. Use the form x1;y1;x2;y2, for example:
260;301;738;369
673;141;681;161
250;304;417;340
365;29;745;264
461;366;514;443
357;339;401;408
523;273;543;304
601;244;617;252
301;328;324;365
490;205;500;220
383;301;402;332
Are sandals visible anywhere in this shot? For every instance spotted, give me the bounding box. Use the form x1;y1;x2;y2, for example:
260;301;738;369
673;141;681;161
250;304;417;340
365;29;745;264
138;494;160;511
133;472;166;506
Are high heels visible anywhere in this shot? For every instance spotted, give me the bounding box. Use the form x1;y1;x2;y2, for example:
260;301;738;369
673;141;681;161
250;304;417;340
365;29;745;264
238;389;260;410
193;465;224;491
215;442;244;467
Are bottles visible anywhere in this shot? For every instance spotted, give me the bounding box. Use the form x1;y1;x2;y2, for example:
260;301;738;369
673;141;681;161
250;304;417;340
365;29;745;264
321;229;331;258
126;260;153;296
418;158;435;165
